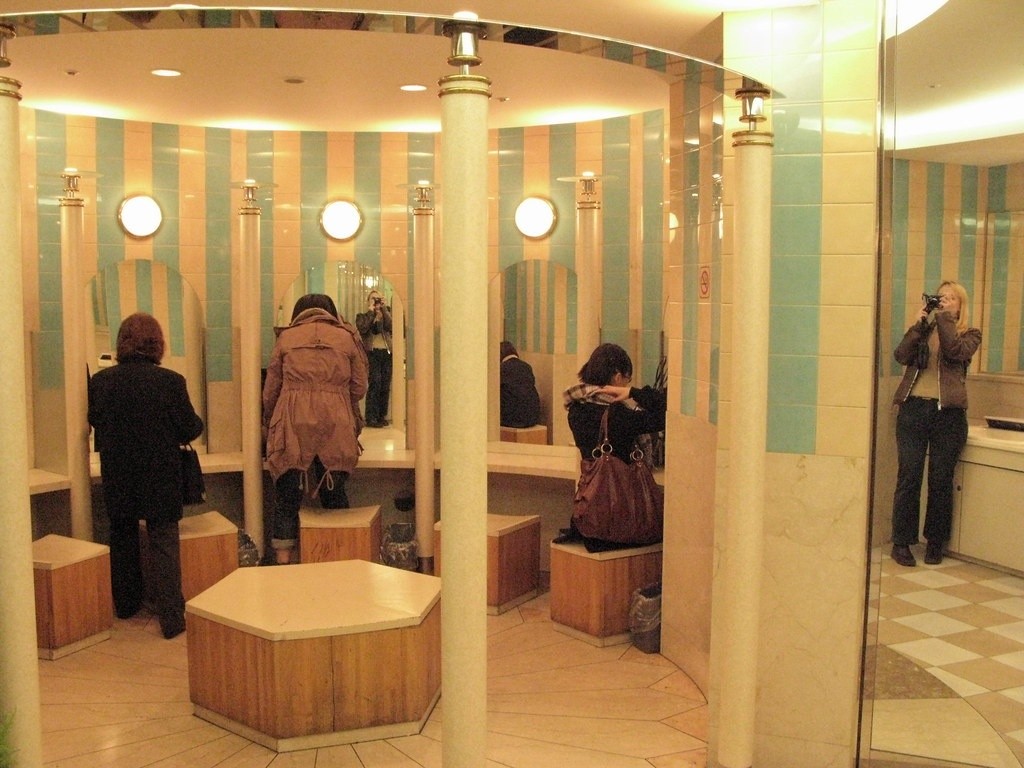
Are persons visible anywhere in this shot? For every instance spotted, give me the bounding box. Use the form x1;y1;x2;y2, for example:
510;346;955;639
86;313;204;639
890;282;983;567
499;340;541;428
356;290;392;428
552;343;666;554
262;293;369;565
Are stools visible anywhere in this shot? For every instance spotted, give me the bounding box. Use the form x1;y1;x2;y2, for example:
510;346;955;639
500;424;548;445
31;534;112;661
433;513;542;616
137;510;240;602
298;505;382;565
549;538;664;648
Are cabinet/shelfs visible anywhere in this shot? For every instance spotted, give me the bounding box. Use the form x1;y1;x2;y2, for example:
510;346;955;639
916;454;1024;578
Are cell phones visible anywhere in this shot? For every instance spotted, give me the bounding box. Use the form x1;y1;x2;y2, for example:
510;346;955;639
372;296;384;304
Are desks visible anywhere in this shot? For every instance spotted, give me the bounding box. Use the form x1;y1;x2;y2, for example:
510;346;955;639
184;559;443;755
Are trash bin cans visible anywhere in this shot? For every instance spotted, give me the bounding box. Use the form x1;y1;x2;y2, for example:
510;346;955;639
385;522;417;572
632;583;661;654
239;530;259;567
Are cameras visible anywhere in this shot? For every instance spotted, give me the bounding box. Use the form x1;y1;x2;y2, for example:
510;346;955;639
921;291;944;309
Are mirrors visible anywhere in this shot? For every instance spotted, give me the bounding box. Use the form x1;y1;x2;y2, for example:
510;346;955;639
974;208;1024;377
277;257;406;451
856;0;1024;768
77;257;207;453
486;258;577;446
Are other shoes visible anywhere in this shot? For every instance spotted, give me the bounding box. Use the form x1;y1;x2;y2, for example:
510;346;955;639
925;542;944;563
365;417;384;428
379;420;389;426
890;544;915;566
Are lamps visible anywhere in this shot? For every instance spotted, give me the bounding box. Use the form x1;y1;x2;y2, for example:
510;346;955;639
118;191;164;242
227;178;279;207
395;179;440;208
555;170;621;200
46;168;104;197
318;201;364;243
514;196;559;242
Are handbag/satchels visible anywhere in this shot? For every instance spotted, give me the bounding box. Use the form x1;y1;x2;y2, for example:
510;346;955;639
572;406;664;541
180;443;206;505
